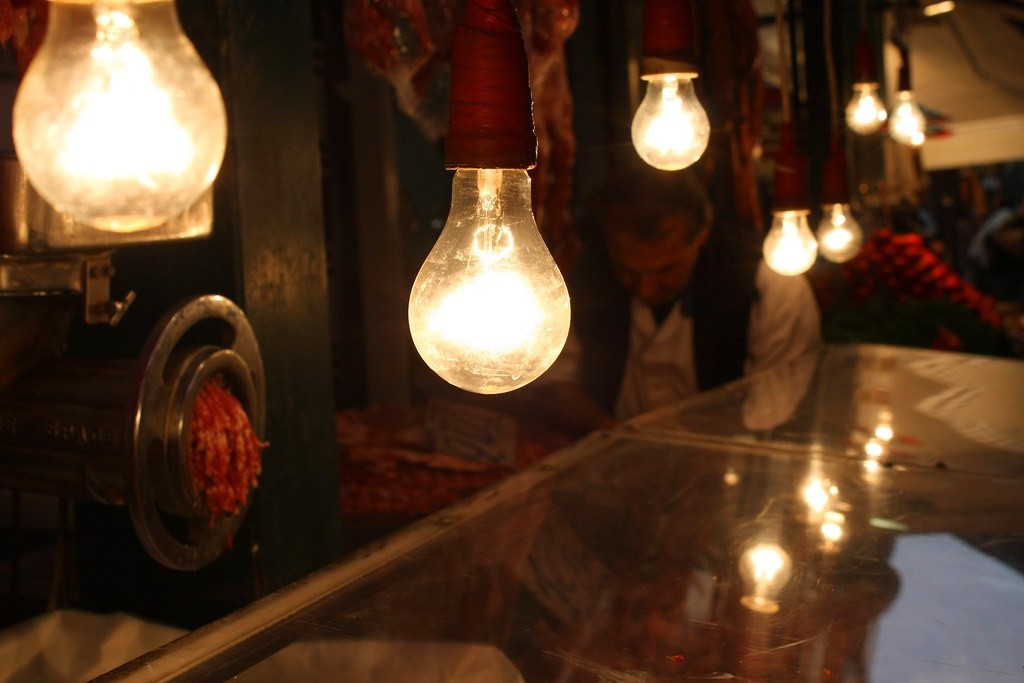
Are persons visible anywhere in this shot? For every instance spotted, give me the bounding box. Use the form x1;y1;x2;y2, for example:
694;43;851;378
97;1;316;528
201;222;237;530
573;161;821;422
